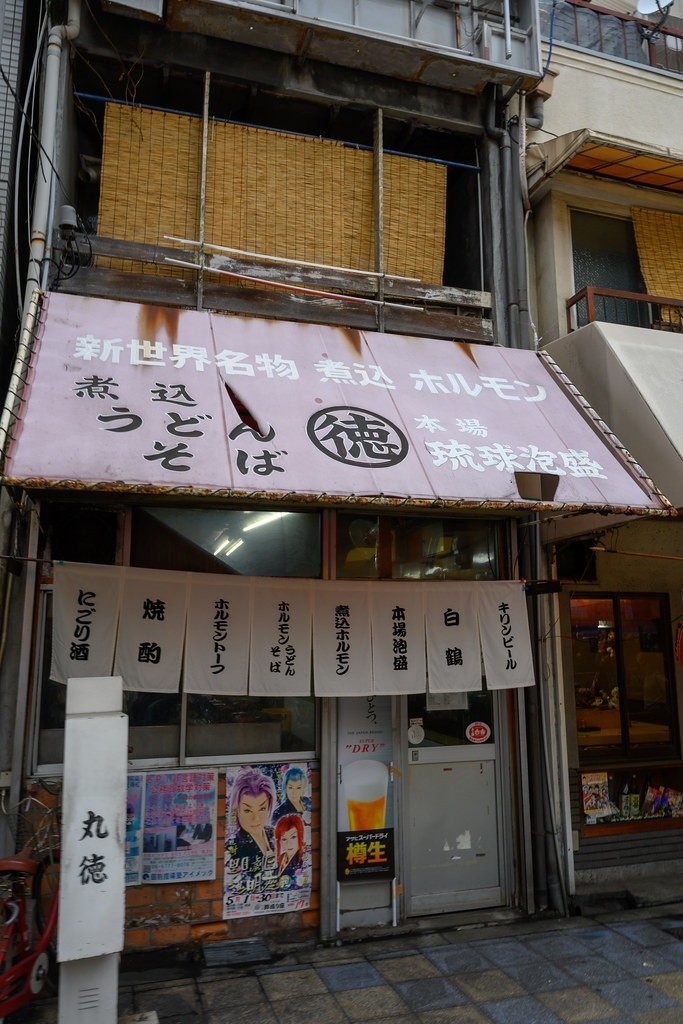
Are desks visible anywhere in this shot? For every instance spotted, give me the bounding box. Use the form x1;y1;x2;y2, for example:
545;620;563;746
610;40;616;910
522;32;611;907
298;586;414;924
578;723;670;746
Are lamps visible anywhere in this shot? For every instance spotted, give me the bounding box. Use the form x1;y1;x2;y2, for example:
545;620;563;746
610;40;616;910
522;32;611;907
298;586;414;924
515;472;560;499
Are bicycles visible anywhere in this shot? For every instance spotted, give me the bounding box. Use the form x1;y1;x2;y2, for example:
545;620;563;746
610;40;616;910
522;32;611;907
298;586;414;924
0;777;62;1024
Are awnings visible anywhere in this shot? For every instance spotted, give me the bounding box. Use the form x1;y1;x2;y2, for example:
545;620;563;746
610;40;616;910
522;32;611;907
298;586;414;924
526;128;683;205
0;292;680;545
540;321;683;511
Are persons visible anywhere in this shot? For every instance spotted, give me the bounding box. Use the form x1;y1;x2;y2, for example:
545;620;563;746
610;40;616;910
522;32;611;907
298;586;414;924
224;765;311;891
